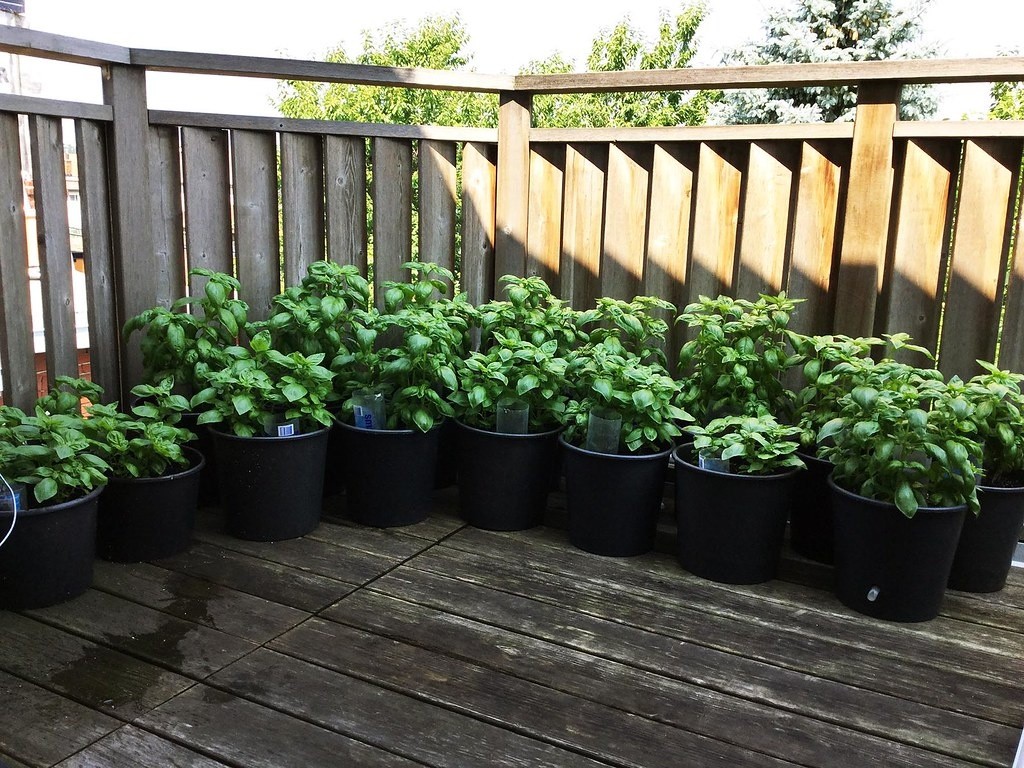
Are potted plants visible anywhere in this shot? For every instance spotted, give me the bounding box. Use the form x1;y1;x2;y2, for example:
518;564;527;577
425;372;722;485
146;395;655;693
1;259;1024;622
